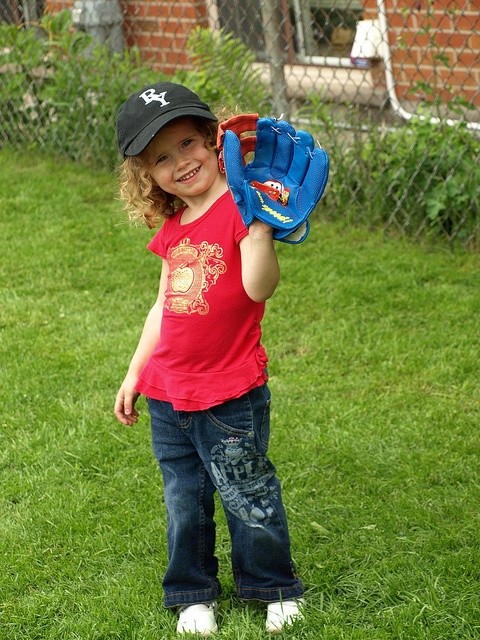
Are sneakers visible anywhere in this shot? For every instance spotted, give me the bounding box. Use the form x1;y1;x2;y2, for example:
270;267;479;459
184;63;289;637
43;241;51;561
265;599;306;634
176;601;217;638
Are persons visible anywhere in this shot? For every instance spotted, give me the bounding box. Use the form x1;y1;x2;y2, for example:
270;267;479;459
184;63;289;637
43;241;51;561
114;81;329;637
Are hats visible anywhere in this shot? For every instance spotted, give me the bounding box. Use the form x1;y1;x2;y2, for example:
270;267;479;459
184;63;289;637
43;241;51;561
115;82;219;161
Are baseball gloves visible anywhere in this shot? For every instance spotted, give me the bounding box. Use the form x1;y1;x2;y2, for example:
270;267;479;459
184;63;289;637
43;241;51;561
217;114;329;244
